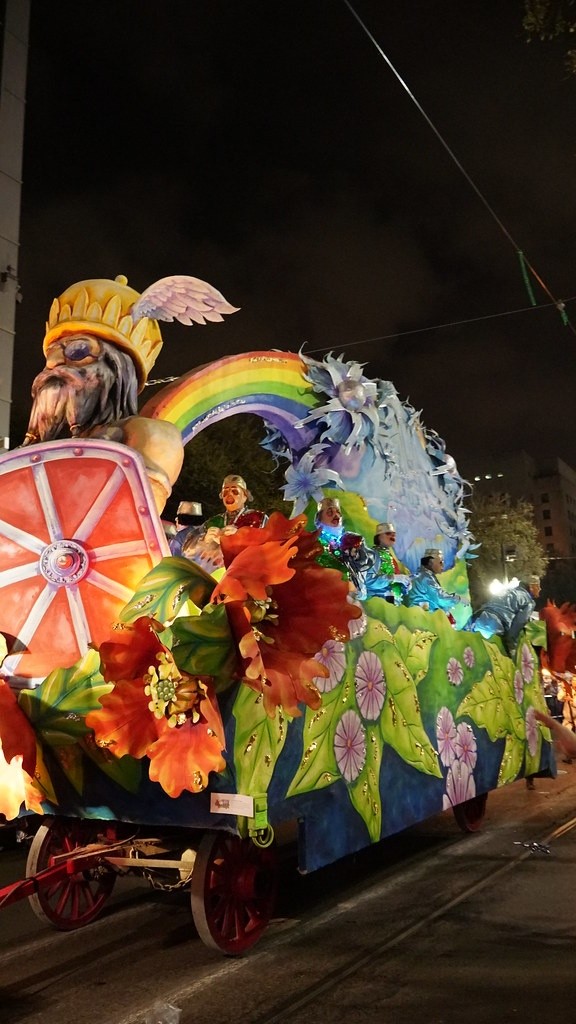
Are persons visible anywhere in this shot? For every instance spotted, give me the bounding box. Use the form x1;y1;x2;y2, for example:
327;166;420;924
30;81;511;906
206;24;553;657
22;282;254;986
310;498;344;552
359;523;412;607
408;549;469;631
525;710;576;790
461;575;540;658
13;274;242;516
170;502;201;557
181;475;269;557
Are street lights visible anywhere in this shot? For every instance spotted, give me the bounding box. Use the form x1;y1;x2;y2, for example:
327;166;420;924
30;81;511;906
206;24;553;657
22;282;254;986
489;514;519;597
